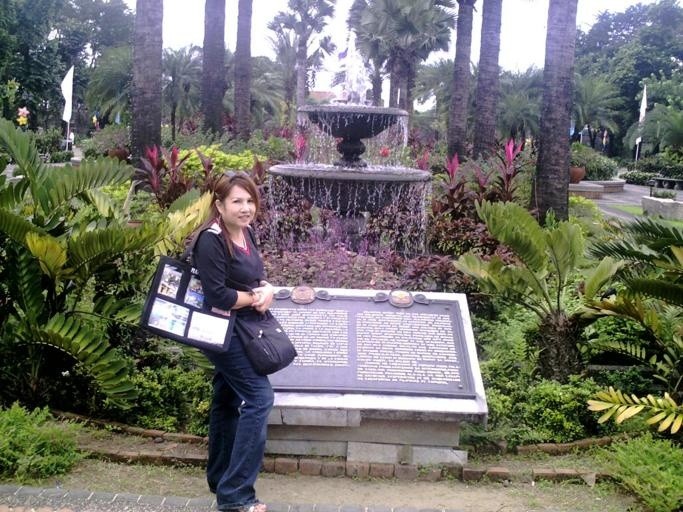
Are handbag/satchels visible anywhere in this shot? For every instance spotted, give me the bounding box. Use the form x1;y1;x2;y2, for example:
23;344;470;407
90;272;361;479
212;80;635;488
233;308;298;377
139;228;237;354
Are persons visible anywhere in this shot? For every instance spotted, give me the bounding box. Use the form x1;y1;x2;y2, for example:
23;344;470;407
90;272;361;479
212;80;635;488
190;171;272;512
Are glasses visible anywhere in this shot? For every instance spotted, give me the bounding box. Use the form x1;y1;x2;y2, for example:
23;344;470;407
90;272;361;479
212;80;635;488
214;170;250;191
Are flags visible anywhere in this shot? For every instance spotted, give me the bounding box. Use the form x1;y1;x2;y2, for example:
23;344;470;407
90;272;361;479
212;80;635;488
634;84;647;145
60;66;73;123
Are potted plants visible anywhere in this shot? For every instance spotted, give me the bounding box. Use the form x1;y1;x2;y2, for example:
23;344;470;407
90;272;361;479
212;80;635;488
570;142;587;184
